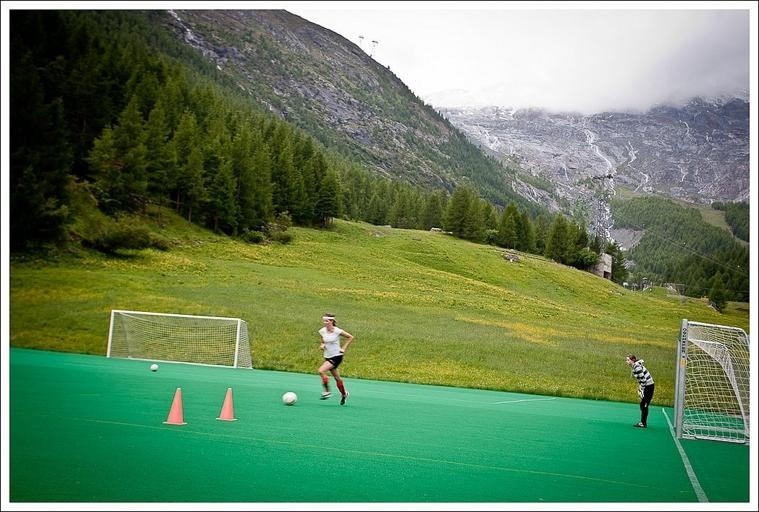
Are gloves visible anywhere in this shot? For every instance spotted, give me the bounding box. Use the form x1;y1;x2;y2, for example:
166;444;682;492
638;388;645;400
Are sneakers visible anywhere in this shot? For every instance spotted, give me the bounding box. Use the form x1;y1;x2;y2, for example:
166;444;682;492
634;422;645;427
323;392;332;398
340;392;349;405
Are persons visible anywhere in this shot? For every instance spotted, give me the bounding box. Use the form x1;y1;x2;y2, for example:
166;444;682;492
318;313;353;406
627;354;655;427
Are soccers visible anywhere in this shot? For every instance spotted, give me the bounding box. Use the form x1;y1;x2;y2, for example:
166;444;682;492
282;392;297;405
151;364;159;371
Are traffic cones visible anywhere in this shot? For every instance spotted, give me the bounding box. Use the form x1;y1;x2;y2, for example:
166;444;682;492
162;387;187;425
216;387;238;422
